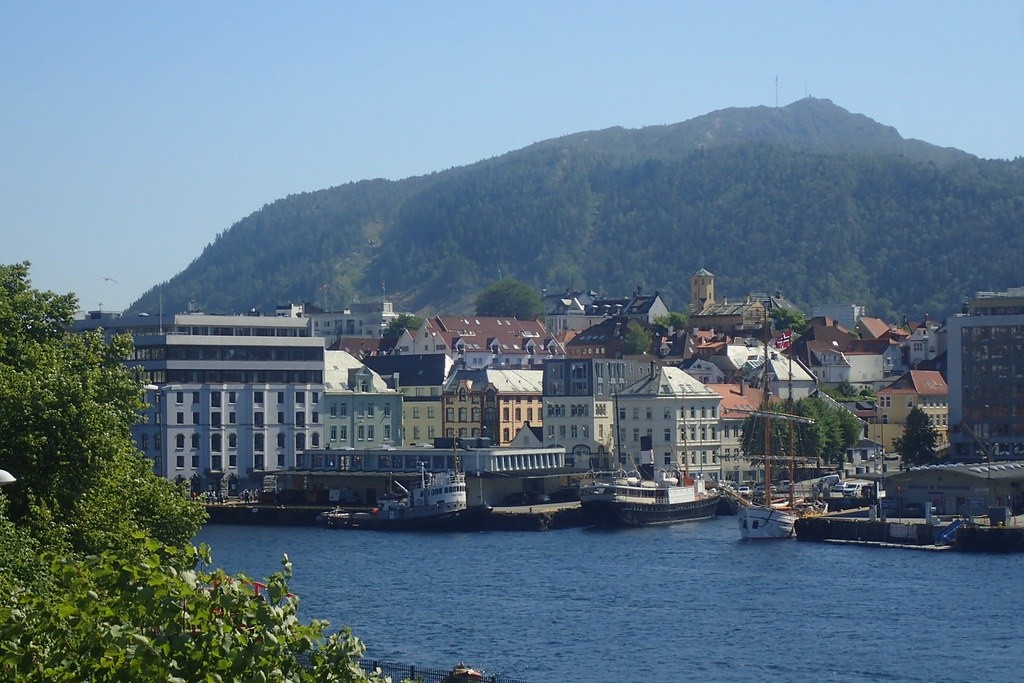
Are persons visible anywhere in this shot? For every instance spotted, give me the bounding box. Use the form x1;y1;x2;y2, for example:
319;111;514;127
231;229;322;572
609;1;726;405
192;490;223;505
822;488;830;511
242;488;261;503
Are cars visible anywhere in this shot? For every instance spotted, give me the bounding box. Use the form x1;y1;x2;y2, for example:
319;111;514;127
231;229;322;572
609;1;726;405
835;482;848;491
738;486;753;496
770;485;777;494
755;486;766;495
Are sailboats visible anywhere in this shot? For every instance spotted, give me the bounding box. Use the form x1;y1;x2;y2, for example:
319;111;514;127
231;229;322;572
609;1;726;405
720;332;829;540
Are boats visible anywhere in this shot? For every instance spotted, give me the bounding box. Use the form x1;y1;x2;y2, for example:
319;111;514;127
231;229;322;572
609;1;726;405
319;463;466;529
503;491;550;506
579;386;722;525
451;662;483;683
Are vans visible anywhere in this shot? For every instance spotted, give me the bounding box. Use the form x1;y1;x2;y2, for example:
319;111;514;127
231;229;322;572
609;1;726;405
844;483;867;498
